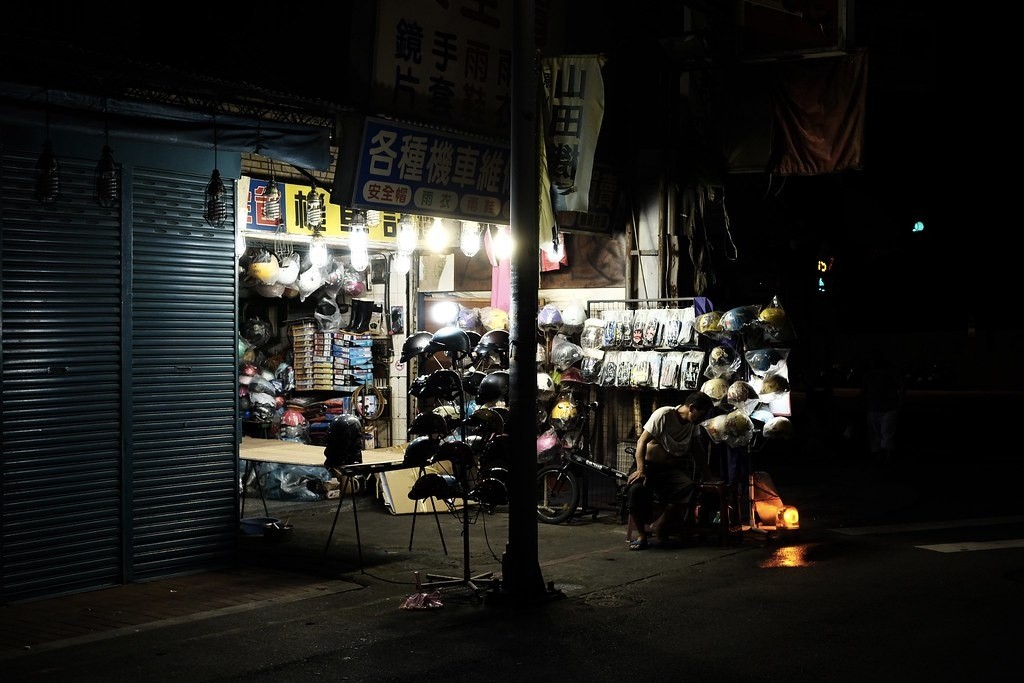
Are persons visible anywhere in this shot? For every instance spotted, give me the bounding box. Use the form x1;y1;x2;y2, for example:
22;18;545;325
623;392;713;551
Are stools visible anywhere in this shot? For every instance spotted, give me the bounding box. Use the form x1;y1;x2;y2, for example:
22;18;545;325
688;479;744;547
627;490;663;541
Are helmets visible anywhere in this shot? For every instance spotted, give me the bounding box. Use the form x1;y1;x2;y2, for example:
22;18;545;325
398;304;584;507
686;304;792;448
240;234;395;500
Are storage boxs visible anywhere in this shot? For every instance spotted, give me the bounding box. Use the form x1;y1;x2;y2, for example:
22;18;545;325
292;325;373;389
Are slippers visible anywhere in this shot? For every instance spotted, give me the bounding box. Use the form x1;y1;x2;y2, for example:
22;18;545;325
629;539;648;550
656;536;668;549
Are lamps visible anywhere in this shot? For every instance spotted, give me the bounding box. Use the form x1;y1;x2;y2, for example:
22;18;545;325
203;143;227;228
307;182;320;226
264;157;279;221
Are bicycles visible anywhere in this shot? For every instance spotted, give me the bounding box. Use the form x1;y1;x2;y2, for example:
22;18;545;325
536;399;640;524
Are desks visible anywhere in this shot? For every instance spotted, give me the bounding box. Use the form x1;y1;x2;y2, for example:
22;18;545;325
239;434;449;575
